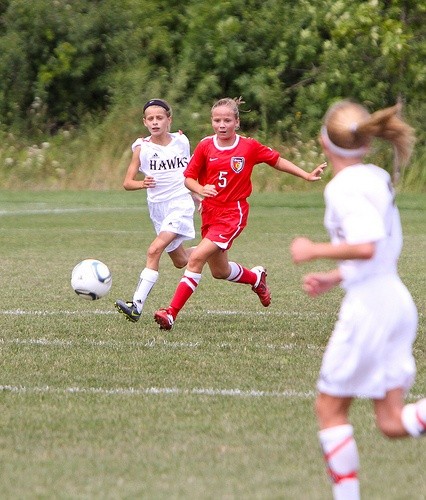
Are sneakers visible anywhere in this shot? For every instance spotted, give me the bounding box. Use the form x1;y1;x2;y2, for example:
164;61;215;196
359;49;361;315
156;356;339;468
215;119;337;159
114;299;142;322
250;266;271;307
153;307;174;332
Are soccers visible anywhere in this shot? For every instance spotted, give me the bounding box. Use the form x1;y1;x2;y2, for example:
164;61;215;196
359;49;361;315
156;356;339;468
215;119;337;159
70;259;113;301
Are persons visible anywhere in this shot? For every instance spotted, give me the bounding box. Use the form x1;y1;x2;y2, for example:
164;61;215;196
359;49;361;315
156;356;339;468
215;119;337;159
114;99;203;323
153;96;328;330
289;99;426;500
